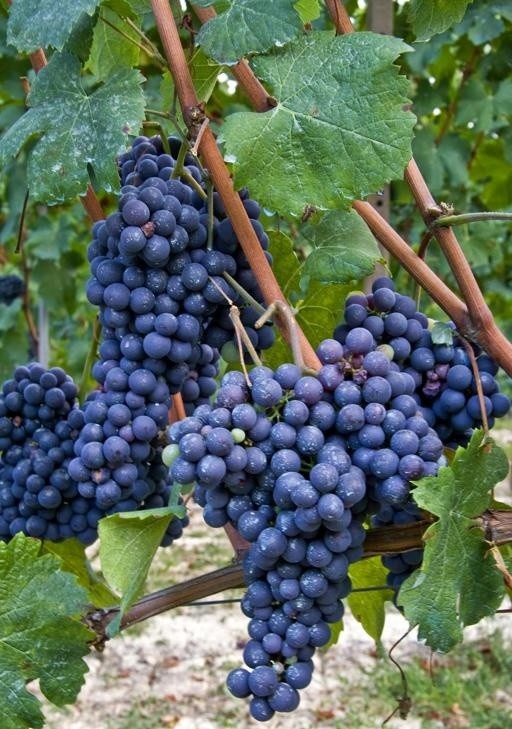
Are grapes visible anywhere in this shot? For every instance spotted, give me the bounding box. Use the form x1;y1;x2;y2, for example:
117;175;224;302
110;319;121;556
0;119;512;721
